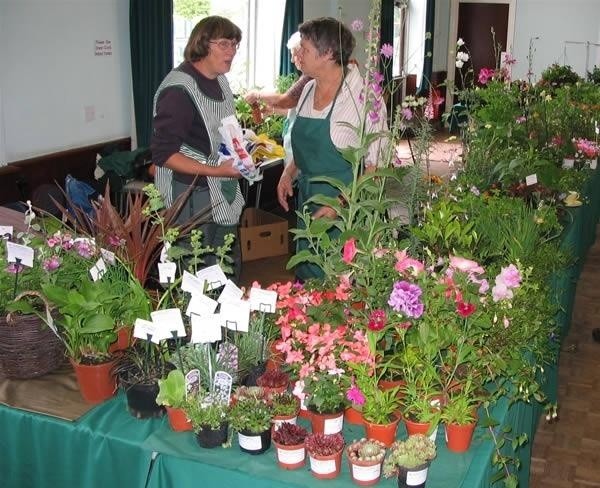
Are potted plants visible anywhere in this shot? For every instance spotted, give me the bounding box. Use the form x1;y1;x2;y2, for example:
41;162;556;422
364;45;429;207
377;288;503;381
40;279;153;405
349;436;387;483
404;348;440;440
237;375;345;480
393;431;436;488
346;362;402;448
117;184;239;448
440;374;478;452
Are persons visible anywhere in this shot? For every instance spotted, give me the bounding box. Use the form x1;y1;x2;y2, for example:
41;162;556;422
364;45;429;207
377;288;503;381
239;32;312;186
150;18;246;300
276;18;390;282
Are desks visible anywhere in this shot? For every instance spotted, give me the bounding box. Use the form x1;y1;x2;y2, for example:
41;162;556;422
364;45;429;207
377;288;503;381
447;102;470;134
237;156;297;209
1;167;599;487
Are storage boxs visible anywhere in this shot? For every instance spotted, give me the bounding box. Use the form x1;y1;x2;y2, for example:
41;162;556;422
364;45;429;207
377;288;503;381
240;206;289;261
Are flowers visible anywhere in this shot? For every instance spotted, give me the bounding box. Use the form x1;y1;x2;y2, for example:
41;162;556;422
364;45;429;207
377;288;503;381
0;204;122;314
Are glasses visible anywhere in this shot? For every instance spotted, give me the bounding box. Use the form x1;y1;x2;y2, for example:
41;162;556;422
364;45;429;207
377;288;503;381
208;40;240;52
